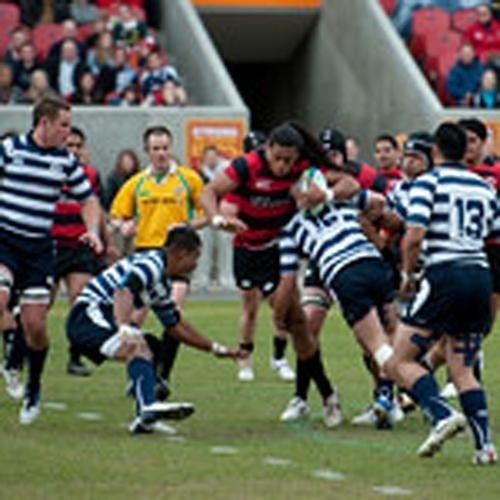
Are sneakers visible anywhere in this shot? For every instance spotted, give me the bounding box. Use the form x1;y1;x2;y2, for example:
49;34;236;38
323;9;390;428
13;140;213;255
417;412;468;457
439;382;458;399
238;360;254;382
472;444;498;465
68;363;90;376
279;387;417;430
0;360;24;401
270;358;296;381
19;381;41;425
124;378;195;436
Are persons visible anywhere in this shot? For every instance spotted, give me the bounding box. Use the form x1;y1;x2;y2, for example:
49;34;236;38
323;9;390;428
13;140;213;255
0;95;499;469
383;0;499;108
0;1;187;105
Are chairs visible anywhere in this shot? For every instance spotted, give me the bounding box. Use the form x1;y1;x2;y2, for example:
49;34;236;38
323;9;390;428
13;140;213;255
410;6;500;109
1;2;190;108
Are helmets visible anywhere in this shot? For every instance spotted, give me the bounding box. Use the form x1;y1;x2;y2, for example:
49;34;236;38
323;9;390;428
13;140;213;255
244;131;266;153
403;132;435;171
319;128;347;163
